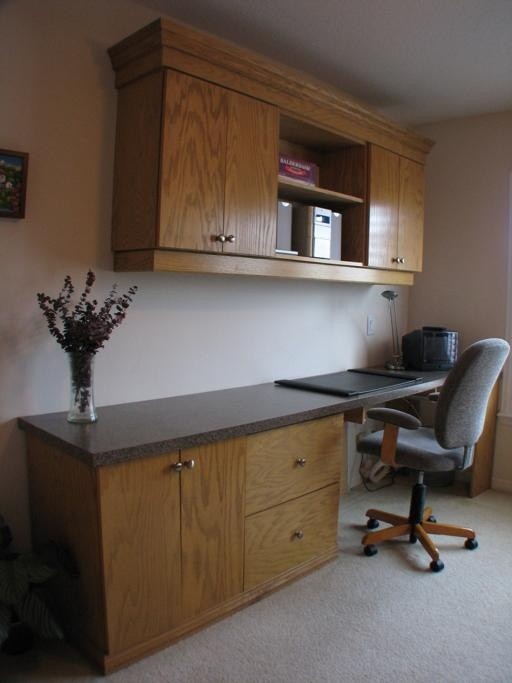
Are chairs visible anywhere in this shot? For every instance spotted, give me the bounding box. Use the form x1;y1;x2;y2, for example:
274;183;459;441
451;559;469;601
355;337;511;572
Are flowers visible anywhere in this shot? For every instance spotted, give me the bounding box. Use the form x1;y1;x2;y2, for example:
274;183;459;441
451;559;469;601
36;267;138;413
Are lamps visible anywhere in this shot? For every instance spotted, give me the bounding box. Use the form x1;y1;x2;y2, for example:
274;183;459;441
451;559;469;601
381;290;406;371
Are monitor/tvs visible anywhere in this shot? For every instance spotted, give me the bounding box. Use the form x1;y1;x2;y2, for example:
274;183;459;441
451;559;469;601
402;330;459;371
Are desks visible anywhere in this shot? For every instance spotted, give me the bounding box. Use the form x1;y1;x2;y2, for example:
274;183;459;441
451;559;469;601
16;365;502;678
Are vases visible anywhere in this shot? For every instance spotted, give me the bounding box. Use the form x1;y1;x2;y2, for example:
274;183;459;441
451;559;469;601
66;351;98;425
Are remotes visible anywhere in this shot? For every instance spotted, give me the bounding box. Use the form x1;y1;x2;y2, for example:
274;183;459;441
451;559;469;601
423;326;447;331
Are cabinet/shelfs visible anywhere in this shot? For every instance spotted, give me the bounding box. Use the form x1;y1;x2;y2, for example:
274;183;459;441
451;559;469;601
106;18;436;285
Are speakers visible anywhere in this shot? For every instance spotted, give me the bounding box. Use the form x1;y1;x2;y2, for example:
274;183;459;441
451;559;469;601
277;201;293;250
330;212;343;259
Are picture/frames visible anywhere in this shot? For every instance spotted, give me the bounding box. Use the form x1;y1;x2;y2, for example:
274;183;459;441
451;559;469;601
0;147;30;220
